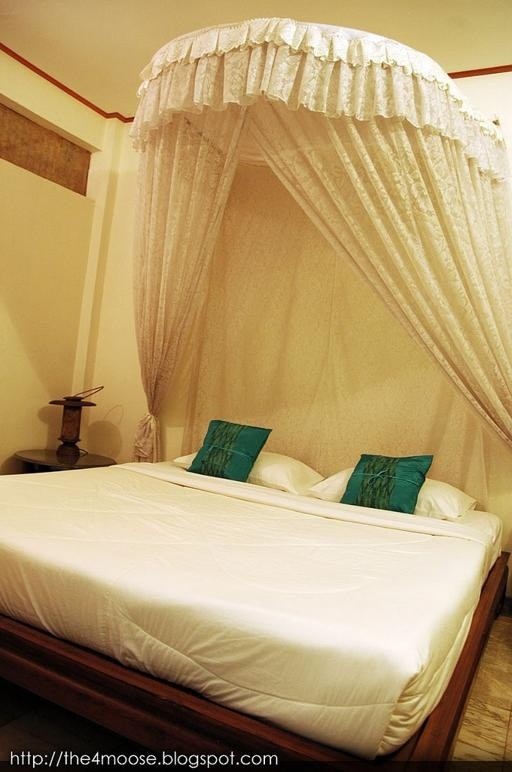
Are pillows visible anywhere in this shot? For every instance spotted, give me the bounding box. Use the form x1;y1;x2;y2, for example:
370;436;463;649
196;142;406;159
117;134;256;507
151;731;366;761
187;419;275;483
309;467;478;522
339;452;433;514
173;451;326;495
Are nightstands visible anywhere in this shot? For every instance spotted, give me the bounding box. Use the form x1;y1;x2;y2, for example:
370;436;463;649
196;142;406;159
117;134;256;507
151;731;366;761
13;449;116;469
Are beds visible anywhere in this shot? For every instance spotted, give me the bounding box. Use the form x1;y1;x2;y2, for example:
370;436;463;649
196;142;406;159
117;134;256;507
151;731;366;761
1;461;511;766
